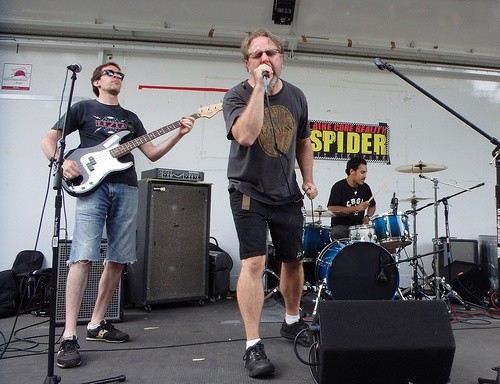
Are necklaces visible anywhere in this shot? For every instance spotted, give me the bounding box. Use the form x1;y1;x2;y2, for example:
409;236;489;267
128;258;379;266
351;185;357;195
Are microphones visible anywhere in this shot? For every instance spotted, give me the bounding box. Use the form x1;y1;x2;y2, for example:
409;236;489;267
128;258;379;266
67;64;82;72
263;63;270;77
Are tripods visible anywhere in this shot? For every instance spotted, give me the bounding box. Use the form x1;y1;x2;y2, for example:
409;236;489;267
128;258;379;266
397;175;470;318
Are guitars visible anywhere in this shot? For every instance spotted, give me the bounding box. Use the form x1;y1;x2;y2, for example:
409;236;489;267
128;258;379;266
61;101;224;198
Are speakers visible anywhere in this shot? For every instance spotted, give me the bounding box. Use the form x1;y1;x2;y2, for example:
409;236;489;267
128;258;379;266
428;260;492;305
436;239;478;266
54;239;123;324
124;178;212;312
308;300;455;384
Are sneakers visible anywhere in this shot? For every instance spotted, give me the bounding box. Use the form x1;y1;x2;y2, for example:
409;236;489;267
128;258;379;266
85;318;129;343
242;339;275;378
279;317;315;347
56;335;82;369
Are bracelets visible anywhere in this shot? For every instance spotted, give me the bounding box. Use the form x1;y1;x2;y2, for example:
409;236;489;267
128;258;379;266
365;215;370;217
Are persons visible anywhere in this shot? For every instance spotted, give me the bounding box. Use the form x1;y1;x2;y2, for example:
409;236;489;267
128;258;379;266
223;29;318;378
41;63;195;369
326;157;376;241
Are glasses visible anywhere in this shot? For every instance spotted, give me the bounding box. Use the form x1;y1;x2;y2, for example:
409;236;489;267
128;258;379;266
247;48;281;59
100;69;125;82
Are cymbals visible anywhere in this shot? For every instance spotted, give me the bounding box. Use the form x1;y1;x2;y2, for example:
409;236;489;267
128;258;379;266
398;197;434;203
303;209;353;218
393;163;449;174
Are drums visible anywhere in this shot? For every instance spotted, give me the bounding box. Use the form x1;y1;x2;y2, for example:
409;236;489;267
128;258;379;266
302;223;332;263
314;238;401;301
369;210;413;250
348;224;380;246
